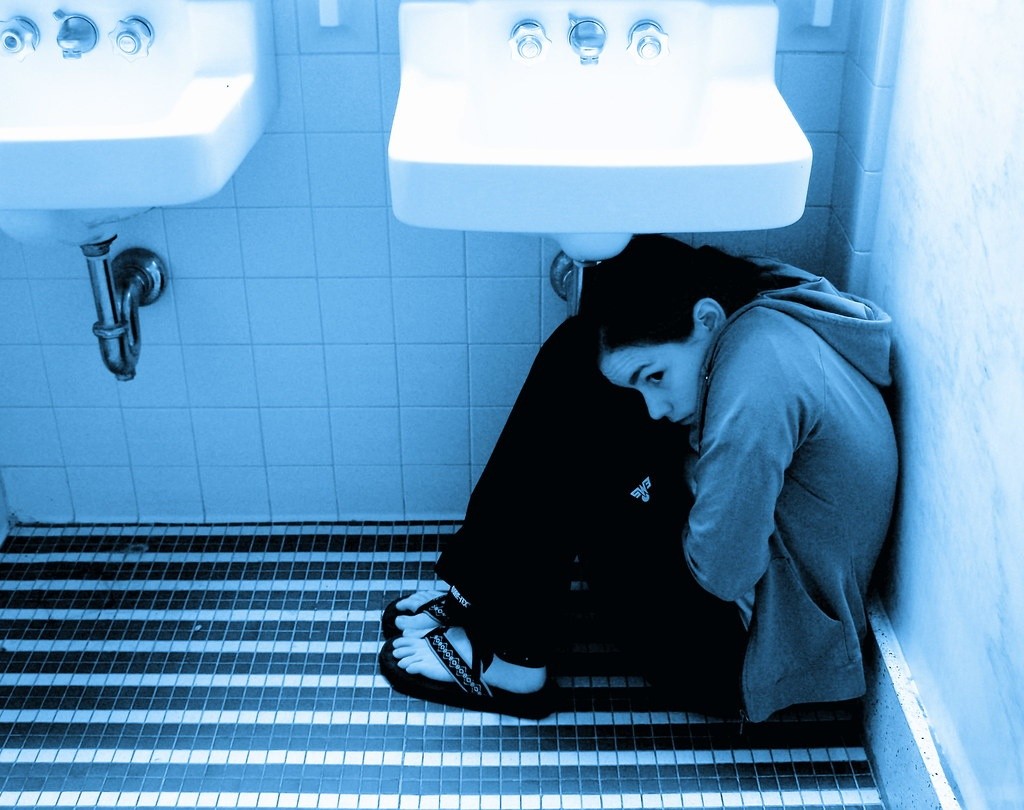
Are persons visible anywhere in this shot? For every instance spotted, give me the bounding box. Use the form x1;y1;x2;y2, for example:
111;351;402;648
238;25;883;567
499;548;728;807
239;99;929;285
378;233;897;725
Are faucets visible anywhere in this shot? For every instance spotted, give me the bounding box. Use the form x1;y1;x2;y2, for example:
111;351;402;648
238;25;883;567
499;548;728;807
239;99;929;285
53;10;98;60
565;21;608;67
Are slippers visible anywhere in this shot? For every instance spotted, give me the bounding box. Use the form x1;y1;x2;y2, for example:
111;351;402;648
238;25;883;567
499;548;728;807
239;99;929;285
379;589;555;718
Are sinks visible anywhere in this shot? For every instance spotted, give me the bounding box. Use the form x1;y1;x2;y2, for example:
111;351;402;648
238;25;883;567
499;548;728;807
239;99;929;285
2;1;280;247
387;0;814;263
